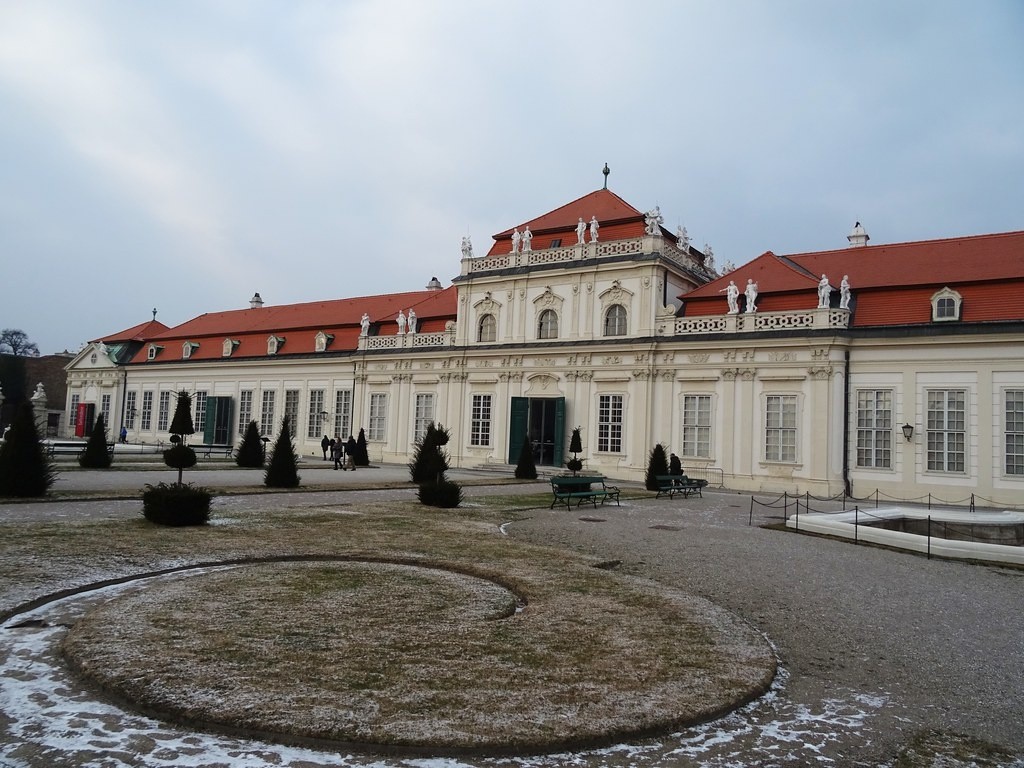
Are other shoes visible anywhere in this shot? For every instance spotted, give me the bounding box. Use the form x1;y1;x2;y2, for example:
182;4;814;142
351;468;356;471
334;468;338;470
343;468;346;471
340;465;343;469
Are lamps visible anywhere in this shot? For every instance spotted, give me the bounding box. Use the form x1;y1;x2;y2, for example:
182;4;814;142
902;423;914;442
320;411;329;422
130;406;138;417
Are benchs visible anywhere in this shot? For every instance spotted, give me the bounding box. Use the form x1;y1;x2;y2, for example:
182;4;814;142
656;475;706;500
49;443;115;462
187;443;234;460
550;477;621;511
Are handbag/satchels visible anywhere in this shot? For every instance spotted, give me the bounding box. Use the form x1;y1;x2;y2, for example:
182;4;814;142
341;452;343;457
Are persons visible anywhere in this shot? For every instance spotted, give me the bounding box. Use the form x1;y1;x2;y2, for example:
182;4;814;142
332;437;343;470
396;308;417;333
744;279;758;311
99;340;108;355
676;225;692;255
511;226;533;251
644;206;664;234
589;216;599;241
343;436;356;471
719;280;739;312
120;427;128;444
575;218;587;243
720;259;735;276
817;274;831;306
321;435;329;461
461;235;475;258
360;312;370;335
839;275;851;309
702;243;716;271
